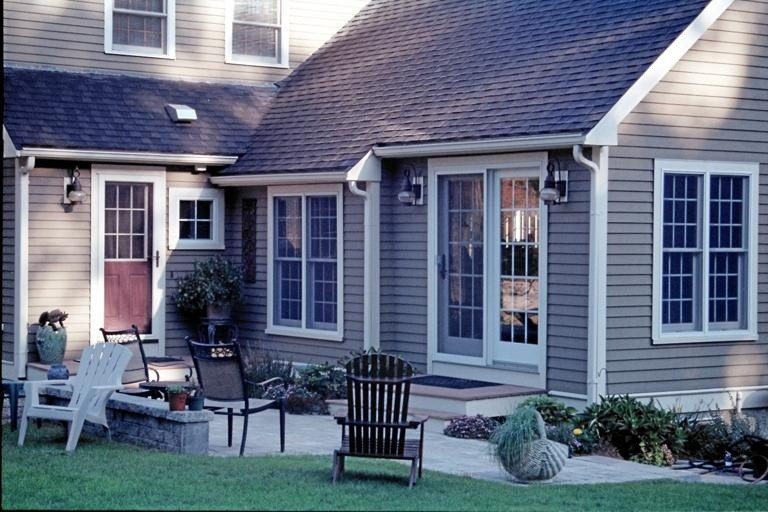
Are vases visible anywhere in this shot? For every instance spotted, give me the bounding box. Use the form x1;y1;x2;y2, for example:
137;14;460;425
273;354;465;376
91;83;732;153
36;313;69;367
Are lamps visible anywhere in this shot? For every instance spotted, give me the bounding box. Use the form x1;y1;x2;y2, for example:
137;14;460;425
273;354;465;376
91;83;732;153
63;165;86;206
397;162;424;206
539;151;569;205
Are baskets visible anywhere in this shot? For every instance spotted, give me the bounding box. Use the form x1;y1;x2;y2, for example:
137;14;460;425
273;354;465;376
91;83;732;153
501;410;569;480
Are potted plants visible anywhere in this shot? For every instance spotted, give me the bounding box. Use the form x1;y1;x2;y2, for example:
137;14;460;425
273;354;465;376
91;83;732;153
172;256;247;325
482;393;569;481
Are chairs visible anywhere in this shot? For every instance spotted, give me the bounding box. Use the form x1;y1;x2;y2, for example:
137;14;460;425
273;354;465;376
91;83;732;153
0;325;286;458
333;352;425;490
